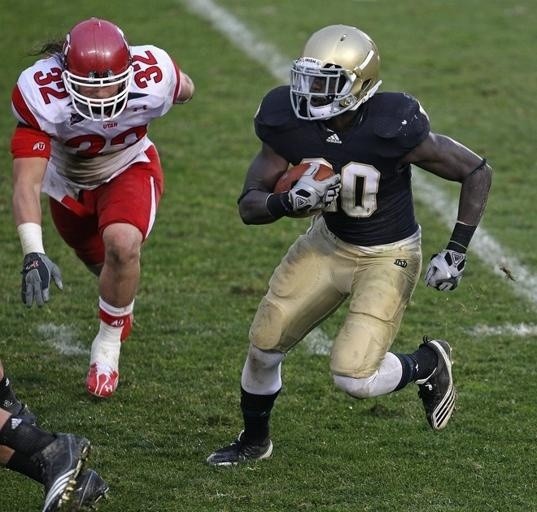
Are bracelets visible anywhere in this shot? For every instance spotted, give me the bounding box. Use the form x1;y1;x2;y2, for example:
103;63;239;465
16;222;45;256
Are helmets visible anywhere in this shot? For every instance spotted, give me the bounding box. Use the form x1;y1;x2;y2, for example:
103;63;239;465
62;15;132;121
292;24;382;121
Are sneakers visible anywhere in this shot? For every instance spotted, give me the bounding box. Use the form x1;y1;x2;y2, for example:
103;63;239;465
84;341;121;399
205;431;275;469
77;466;110;509
415;340;458;431
33;430;92;511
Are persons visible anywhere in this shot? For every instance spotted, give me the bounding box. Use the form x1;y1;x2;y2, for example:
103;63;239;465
0;409;112;511
0;359;38;428
207;25;495;469
10;16;194;397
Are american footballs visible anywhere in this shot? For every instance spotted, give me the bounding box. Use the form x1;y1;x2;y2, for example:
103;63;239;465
274;163;335;218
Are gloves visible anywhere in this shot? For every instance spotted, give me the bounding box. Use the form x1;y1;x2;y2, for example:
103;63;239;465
277;162;344;212
424;250;468;290
22;251;64;309
